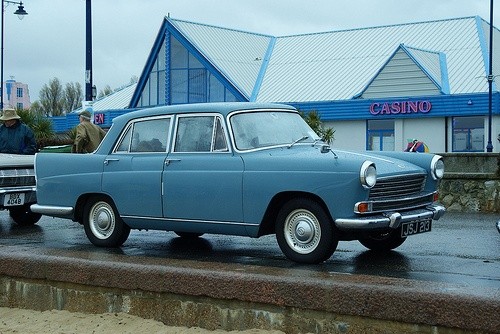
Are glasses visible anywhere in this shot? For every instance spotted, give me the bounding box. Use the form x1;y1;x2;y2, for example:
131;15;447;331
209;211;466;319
78;117;80;119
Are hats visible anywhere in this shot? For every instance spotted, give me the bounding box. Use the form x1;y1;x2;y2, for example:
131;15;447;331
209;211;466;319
78;110;91;118
0;109;21;120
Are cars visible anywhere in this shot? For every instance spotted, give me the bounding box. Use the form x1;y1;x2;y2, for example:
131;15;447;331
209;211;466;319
0;153;42;225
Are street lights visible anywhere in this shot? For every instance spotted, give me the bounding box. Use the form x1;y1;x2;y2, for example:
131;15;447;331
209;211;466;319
0;0;28;113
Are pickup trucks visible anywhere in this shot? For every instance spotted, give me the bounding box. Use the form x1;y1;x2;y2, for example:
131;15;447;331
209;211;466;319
30;102;447;265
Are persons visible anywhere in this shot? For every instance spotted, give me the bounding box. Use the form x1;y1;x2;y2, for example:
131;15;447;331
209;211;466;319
73;111;107;153
0;109;37;155
